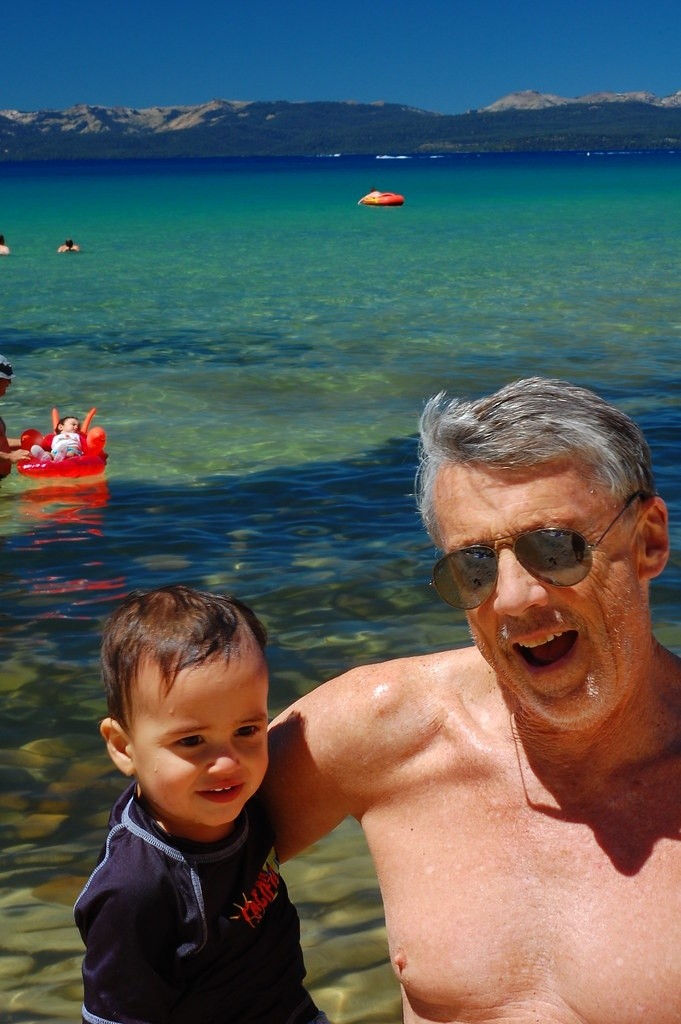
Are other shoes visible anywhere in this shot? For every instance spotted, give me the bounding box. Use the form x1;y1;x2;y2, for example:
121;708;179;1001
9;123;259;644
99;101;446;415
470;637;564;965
31;445;53;463
54;444;68;461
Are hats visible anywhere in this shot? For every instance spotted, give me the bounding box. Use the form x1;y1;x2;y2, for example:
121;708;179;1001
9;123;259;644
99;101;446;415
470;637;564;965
0;355;17;380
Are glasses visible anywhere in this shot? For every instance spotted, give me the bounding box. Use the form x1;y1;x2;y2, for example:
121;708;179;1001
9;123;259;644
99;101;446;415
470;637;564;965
428;490;648;610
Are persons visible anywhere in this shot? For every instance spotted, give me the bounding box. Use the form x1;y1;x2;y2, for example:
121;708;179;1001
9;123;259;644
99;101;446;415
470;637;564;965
357;186;381;204
0;234;9;256
30;416;82;462
72;584;334;1024
64;239;76;251
0;354;44;479
252;374;681;1024
